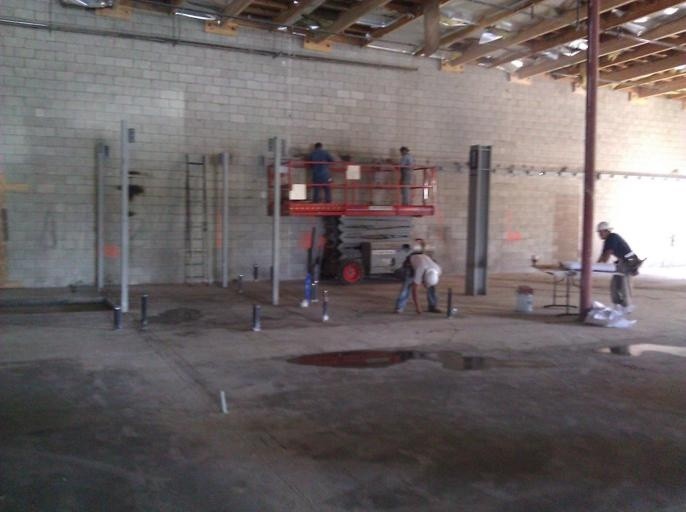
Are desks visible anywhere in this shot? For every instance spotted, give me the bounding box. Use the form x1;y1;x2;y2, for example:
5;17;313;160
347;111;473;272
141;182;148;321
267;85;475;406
533;264;625;317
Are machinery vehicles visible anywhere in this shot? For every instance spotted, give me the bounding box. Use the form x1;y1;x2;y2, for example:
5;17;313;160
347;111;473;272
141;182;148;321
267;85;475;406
268;159;437;286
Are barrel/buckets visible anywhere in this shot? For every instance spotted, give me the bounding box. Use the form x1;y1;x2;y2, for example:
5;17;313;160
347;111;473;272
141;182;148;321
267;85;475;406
514;286;534;313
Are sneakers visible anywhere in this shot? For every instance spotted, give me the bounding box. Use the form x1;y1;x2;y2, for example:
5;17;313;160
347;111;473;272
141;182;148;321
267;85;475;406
394;307;403;313
429;308;443;312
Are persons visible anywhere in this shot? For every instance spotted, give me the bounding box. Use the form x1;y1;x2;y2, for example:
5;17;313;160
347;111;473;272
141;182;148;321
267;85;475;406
396;146;414;206
597;221;639;309
394;251;443;315
309;142;337;202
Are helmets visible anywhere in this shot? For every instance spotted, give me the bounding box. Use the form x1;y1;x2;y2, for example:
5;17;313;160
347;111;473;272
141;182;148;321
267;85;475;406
597;222;608;231
424;269;439;288
